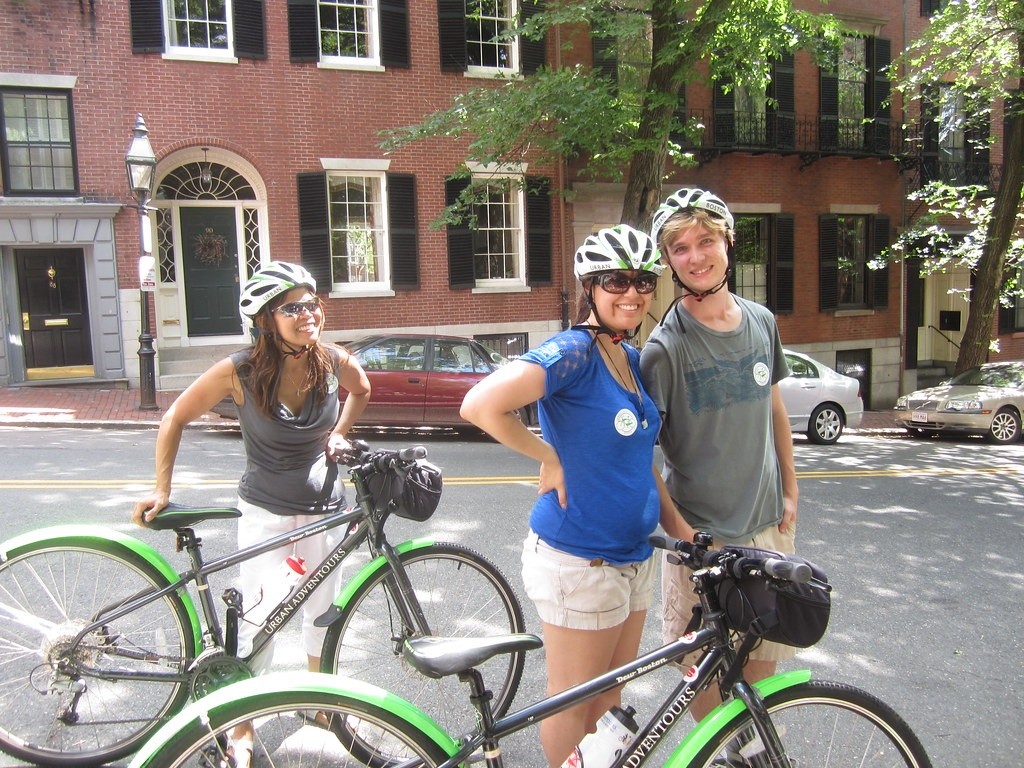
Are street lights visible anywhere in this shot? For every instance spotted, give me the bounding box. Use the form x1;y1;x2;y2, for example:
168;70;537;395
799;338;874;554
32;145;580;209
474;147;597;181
121;113;163;409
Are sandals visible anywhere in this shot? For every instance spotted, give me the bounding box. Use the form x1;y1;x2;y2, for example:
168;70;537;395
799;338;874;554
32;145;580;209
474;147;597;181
298;710;331;730
227;736;254;768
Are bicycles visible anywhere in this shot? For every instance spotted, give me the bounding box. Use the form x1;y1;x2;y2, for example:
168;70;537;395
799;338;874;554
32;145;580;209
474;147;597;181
0;440;529;767
127;528;935;768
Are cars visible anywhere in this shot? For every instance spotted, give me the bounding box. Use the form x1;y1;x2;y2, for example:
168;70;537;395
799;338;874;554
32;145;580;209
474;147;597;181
210;333;540;446
776;349;864;445
893;359;1023;442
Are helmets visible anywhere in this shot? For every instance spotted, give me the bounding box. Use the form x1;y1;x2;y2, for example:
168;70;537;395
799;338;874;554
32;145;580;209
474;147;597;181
239;261;318;359
650;188;734;302
574;223;666;345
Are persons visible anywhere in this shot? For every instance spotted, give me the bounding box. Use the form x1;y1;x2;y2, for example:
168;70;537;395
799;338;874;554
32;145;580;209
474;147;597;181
130;261;372;768
638;184;808;759
457;224;666;766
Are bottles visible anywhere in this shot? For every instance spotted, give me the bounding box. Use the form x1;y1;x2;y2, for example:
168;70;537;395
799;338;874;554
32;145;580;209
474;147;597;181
554;705;639;768
242;556;306;627
